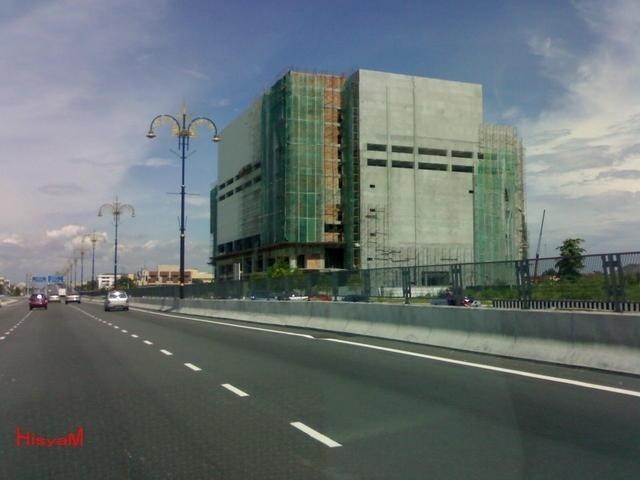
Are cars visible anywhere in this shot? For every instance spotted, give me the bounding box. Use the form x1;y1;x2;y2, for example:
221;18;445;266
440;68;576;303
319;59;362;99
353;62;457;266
28;291;81;311
429;287;478;307
230;291;371;302
101;289;130;312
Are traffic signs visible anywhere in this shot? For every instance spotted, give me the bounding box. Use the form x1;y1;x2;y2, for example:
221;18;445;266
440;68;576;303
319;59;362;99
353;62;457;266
30;276;47;283
48;275;65;284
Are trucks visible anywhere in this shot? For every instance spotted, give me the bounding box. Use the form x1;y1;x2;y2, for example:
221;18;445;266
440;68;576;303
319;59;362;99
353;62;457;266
57;287;67;297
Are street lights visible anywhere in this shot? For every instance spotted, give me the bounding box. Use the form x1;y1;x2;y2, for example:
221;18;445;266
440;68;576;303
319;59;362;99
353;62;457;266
143;105;222;304
60;227;106;292
94;192;136;291
